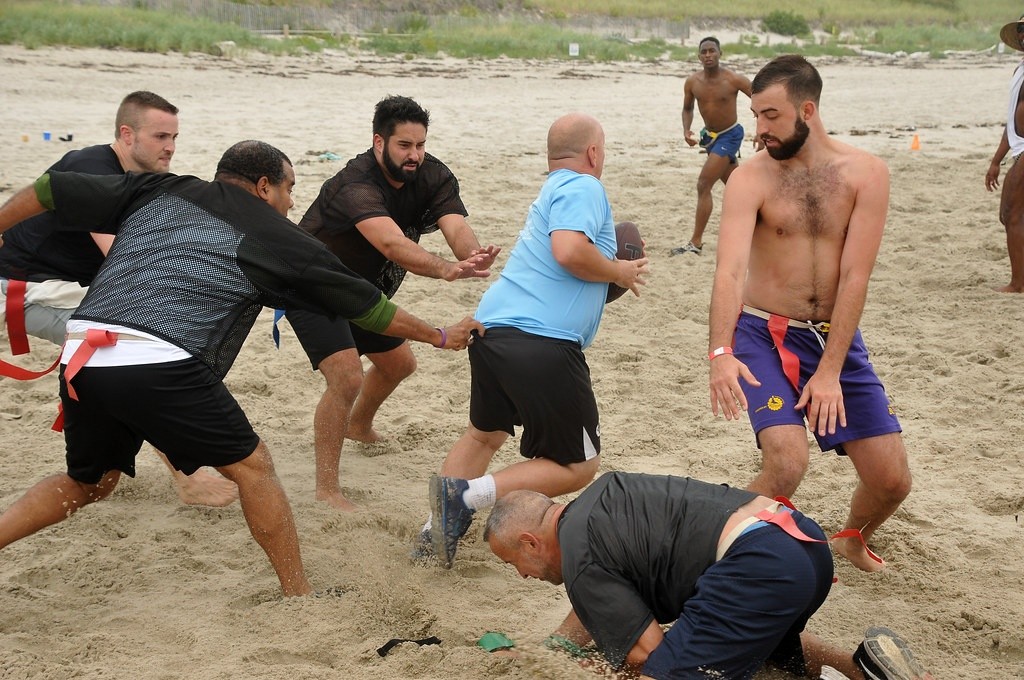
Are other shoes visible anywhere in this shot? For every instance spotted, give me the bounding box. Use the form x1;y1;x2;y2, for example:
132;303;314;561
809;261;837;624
818;665;850;680
854;626;936;680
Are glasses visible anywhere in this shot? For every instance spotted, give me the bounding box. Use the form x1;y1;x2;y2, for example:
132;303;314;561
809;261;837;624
1017;25;1024;33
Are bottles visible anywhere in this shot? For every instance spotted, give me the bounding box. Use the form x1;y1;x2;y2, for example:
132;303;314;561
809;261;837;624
911;132;920;150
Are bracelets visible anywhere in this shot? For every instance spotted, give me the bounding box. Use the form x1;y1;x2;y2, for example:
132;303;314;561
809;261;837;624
710;347;733;362
434;327;447;348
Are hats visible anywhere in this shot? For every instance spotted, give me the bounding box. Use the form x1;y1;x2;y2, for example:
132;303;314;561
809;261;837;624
1000;15;1024;52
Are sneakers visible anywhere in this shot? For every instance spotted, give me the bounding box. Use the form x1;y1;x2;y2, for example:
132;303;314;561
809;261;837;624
670;241;705;256
411;528;433;569
428;475;476;571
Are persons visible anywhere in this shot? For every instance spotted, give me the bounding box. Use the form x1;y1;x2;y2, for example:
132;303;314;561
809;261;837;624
411;113;650;569
484;472;924;680
670;37;764;256
0;140;485;598
0;91;239;507
285;94;501;513
709;54;912;572
985;15;1024;293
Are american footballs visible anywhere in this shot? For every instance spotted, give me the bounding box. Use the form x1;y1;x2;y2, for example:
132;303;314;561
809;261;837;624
605;221;644;304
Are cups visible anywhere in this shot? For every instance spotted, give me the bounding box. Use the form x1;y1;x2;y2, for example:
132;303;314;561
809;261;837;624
44;132;50;139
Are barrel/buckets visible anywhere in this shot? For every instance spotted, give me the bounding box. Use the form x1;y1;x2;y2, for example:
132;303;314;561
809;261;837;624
569;42;579;57
